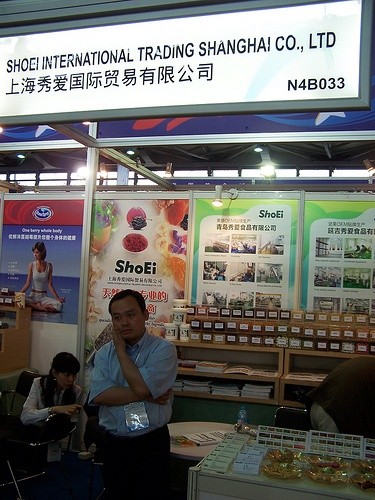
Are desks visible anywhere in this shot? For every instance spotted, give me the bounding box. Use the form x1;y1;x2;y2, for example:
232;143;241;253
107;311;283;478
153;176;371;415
167;421;235;460
187;440;375;500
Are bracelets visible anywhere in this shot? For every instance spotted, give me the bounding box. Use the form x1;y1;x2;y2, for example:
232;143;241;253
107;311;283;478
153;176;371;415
49;406;52;415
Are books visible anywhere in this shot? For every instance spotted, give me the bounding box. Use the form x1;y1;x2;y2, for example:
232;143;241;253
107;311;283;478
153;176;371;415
182;429;227;446
171;360;279;399
286;373;328;381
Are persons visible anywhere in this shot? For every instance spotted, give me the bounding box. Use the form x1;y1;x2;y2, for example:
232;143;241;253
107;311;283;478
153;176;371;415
15;352;83;476
20;241;66;313
87;289;178;500
305;356;375;434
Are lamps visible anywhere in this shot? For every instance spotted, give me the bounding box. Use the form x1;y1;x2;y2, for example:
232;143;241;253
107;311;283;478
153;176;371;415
363;159;375;176
212;184;238;207
254;143;264;152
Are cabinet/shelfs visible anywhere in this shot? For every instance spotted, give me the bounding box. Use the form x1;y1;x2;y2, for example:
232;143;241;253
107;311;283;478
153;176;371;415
278;348;375;408
171;340;285;404
0;305;31;375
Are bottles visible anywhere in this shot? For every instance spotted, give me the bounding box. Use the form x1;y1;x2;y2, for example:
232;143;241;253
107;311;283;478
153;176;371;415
237;407;247;423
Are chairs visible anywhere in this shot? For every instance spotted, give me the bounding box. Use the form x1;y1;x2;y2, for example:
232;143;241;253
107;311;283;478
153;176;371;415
0;370;76;500
272;407;318;431
